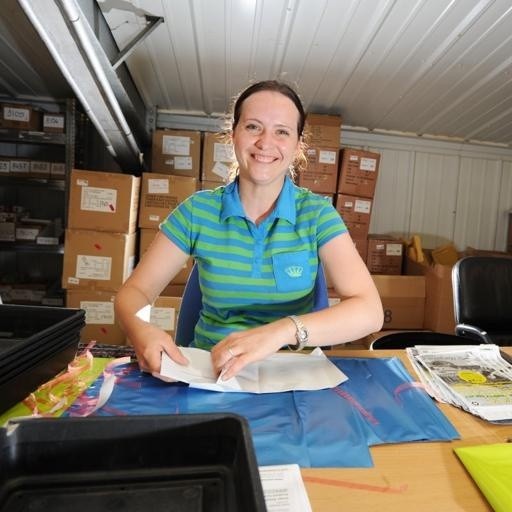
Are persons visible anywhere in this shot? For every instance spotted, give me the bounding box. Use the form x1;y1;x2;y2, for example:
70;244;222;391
114;81;384;382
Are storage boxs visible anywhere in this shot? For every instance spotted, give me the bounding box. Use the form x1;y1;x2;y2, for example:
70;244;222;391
0;102;65;311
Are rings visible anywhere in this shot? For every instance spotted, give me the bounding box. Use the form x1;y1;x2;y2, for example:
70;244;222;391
228;348;234;357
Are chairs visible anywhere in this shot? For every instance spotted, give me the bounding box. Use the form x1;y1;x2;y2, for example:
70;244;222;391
369;332;478;350
175;257;333;349
451;254;512;346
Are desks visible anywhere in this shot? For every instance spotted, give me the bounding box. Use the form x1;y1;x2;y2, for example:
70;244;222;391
1;343;511;511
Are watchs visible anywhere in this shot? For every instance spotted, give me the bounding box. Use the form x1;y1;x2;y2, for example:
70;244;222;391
288;315;309;353
